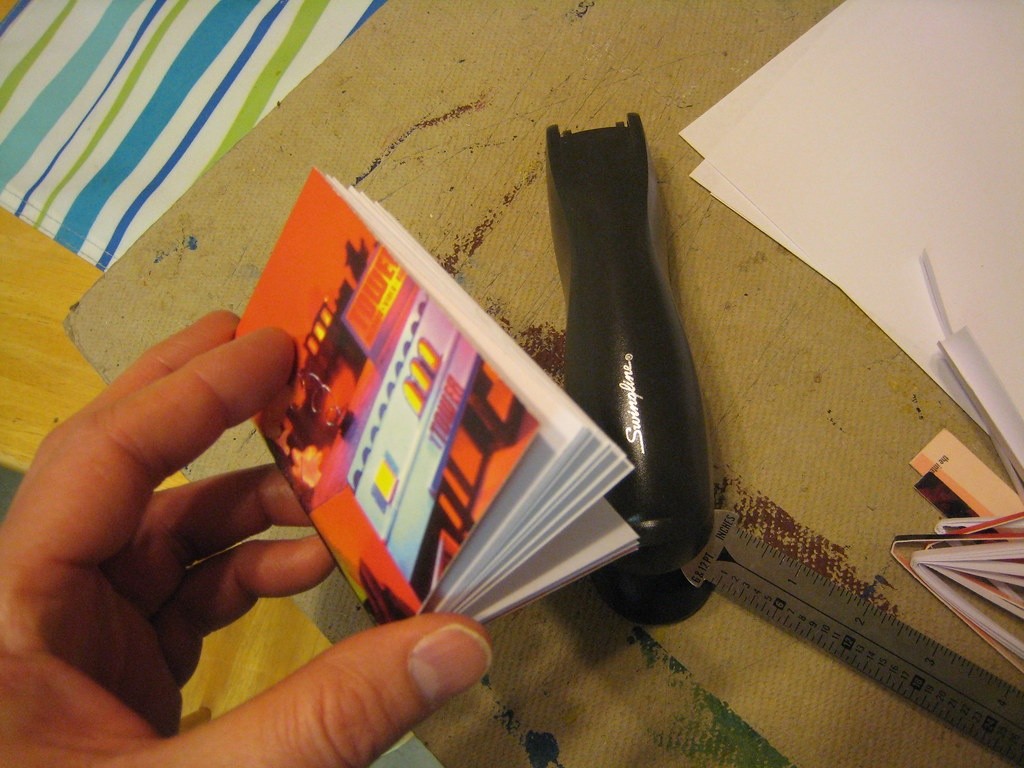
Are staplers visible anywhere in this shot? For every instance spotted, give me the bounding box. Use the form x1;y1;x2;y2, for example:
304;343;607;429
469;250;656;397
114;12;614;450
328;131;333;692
537;110;714;625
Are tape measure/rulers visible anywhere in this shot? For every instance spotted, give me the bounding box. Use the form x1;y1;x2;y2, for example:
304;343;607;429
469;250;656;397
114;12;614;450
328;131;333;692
679;510;1024;767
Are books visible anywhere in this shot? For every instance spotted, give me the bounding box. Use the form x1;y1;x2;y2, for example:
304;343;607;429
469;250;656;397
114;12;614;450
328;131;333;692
232;166;641;625
